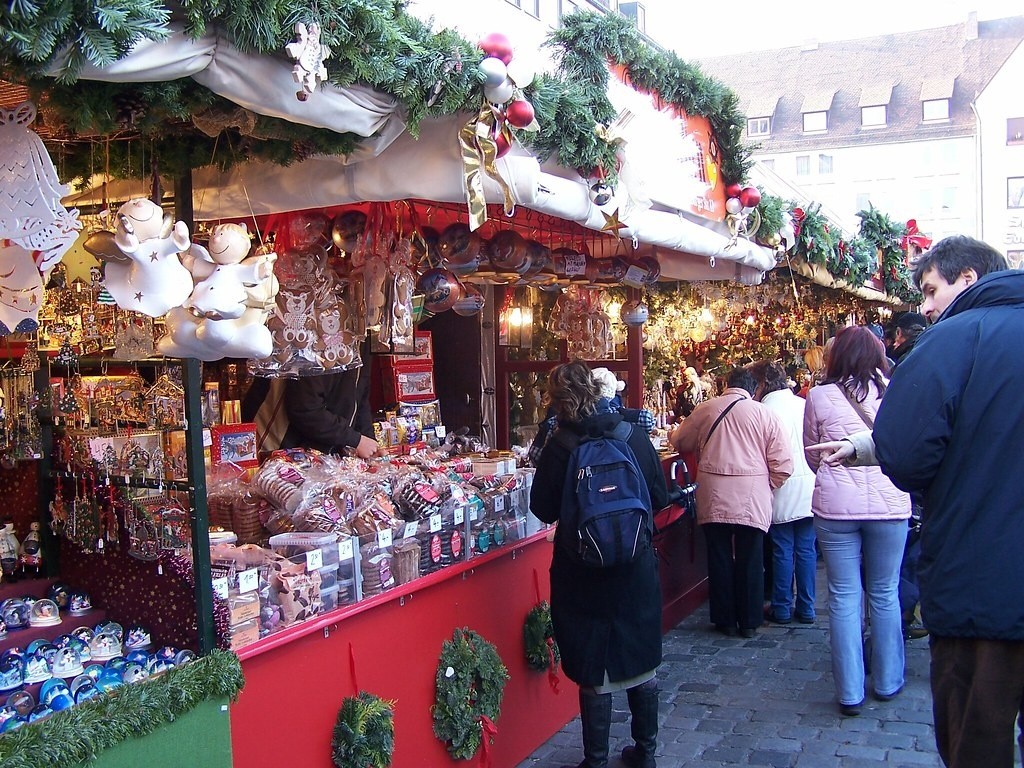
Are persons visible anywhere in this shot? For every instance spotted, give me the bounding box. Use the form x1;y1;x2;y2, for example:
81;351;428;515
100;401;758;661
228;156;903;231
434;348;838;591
528;355;673;768
671;311;930;715
278;330;380;460
871;233;1024;768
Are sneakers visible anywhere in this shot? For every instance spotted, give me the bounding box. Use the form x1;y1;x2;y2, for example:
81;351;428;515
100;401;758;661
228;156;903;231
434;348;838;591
763;605;791;623
794;610;814;624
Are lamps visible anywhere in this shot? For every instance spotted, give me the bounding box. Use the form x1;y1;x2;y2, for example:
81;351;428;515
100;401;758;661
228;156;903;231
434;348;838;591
499;306;534;349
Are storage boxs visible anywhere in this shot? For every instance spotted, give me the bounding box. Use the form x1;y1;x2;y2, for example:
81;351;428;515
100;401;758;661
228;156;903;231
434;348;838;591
163;422;260;480
473;459;517;476
210;527;364;648
370;330;443;434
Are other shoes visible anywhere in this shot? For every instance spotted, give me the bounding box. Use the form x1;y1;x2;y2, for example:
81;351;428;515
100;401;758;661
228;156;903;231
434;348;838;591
841;695;866;716
873;680;907;700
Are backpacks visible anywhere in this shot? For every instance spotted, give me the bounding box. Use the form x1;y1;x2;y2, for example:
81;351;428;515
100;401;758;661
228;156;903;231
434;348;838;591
551;419;655;571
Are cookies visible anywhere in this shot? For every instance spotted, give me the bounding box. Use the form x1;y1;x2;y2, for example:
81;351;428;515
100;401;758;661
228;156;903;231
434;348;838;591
208;465;386;595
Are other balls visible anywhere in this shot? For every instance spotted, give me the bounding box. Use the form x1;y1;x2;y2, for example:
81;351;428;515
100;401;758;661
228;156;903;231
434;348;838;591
291;210;661;328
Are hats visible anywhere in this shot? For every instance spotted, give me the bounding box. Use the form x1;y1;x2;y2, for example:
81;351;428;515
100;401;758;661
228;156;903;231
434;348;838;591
896;312;927;330
589;367;626;403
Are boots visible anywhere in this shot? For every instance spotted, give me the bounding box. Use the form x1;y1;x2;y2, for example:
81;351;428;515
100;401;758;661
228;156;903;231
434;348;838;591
622;684;659;768
577;690;612;768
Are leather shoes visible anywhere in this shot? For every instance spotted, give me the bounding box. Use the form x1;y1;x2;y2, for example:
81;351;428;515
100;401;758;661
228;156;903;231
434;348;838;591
716;623;737;636
742;629;755;638
